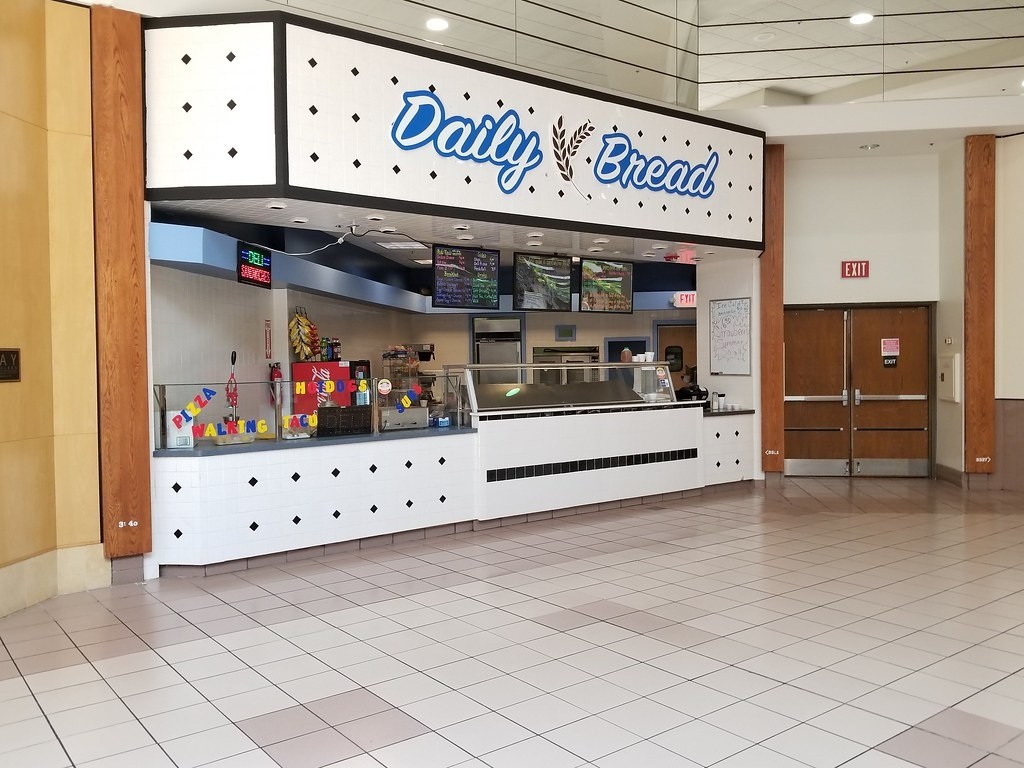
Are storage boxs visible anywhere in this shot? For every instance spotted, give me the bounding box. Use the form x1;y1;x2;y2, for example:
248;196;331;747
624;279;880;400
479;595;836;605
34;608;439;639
209;433;256;446
154;410;198;449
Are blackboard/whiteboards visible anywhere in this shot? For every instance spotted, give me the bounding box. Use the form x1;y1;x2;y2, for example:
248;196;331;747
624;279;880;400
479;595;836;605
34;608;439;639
316;406;374;438
708;296;754;377
512;251;573;312
432;244;501;309
580;258;634;314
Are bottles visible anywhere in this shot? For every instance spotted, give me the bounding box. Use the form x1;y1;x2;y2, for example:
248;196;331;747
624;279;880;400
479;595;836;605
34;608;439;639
320;336;341;362
712;392;719;409
621;347;632;362
718;394;725;409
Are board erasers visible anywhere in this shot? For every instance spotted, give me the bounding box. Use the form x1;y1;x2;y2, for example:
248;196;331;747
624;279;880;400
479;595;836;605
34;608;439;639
711;372;724;376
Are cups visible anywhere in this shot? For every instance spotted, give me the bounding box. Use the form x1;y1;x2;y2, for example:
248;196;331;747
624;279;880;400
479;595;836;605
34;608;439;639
637;354;646;363
733;404;740;410
726;404;733;410
645;352;655;362
632;356;637;362
420;400;428;407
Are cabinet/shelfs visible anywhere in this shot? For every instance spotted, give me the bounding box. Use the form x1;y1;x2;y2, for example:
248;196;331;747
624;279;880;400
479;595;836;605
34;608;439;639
384;359;419;407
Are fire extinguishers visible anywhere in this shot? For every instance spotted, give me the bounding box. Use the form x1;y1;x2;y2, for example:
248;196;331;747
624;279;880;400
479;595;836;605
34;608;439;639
268;362;283;405
